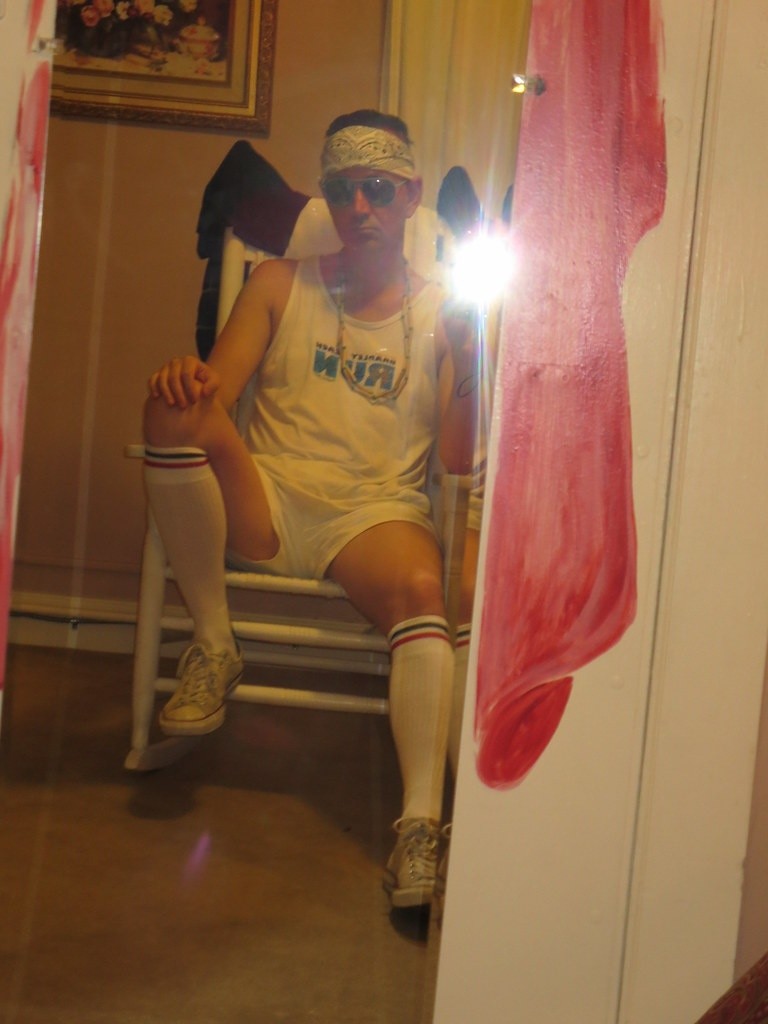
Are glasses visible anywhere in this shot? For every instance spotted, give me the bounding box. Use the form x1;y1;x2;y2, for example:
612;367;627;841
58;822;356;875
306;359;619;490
320;176;408;207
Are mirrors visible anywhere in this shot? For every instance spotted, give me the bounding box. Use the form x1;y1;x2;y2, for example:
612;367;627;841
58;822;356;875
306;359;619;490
0;0;531;1024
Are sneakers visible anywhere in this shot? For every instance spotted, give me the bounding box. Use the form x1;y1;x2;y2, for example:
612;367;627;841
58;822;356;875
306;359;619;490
381;819;440;907
158;639;245;738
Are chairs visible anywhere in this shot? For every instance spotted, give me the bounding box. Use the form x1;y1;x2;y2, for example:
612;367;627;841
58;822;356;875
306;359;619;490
121;226;474;774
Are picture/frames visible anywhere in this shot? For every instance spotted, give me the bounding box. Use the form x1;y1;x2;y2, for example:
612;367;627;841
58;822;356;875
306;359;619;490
49;1;278;134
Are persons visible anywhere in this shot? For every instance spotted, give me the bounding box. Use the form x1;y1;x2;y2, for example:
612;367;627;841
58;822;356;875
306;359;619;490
143;107;488;910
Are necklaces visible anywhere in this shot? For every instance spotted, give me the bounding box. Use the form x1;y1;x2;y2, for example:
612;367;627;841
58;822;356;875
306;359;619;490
333;252;414;402
